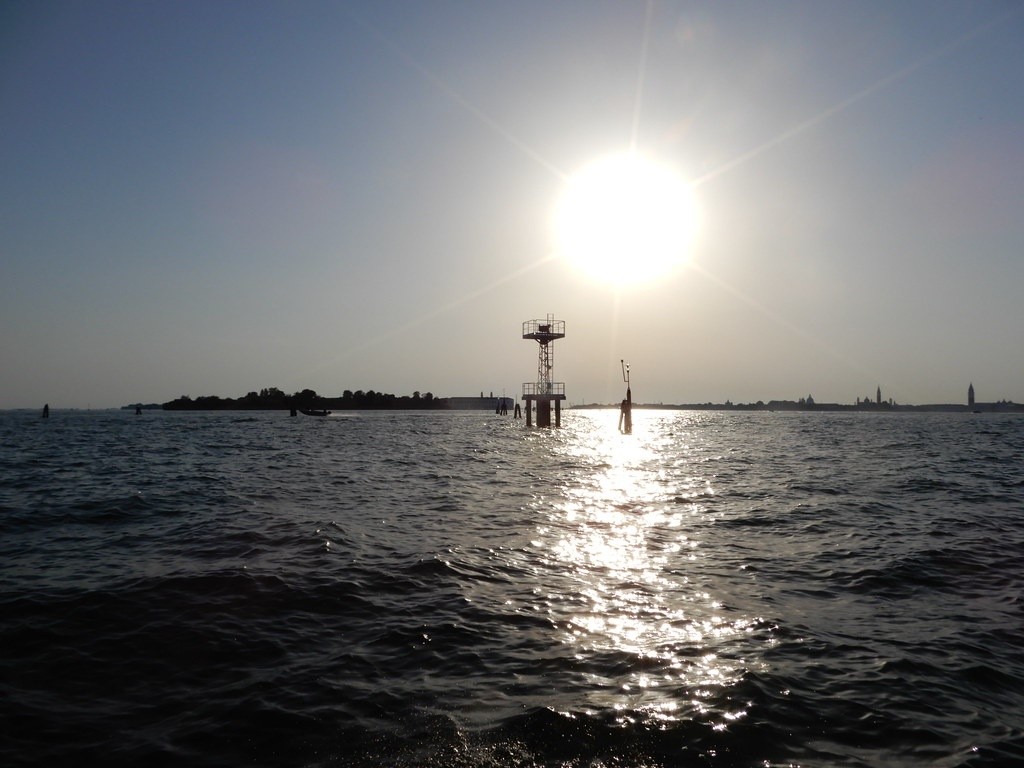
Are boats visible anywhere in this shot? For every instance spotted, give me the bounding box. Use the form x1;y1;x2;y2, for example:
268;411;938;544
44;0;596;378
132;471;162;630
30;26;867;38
299;407;332;417
